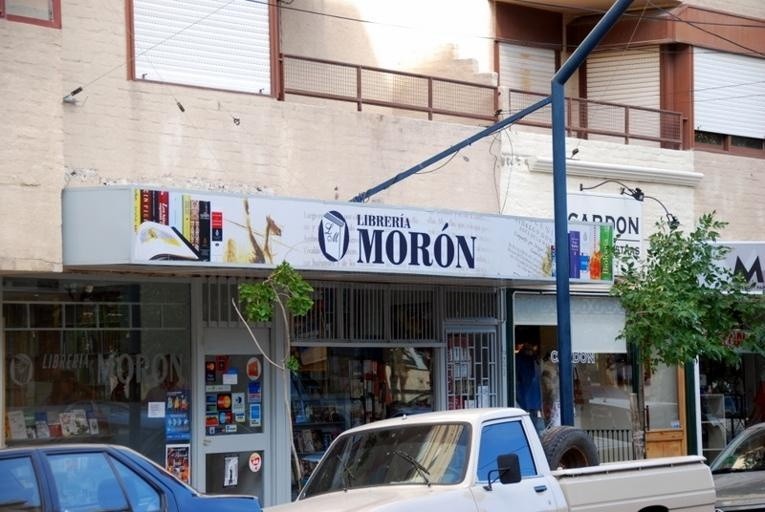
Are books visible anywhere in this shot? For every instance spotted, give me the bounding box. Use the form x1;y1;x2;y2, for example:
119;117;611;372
4;408;99;440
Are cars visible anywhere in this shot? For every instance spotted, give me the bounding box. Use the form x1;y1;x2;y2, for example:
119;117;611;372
66;401;163;432
1;444;262;512
711;422;765;511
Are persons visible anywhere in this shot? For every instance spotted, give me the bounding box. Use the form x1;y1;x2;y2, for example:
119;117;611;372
514;335;542;426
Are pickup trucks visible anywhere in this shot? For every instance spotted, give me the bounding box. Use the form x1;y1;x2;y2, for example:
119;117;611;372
262;406;716;512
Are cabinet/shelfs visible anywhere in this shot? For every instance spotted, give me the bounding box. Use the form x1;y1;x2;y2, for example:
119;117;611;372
700;394;726;464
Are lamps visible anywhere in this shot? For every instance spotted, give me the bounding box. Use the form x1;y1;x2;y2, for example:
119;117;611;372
621;188;680;230
581;181;644;201
